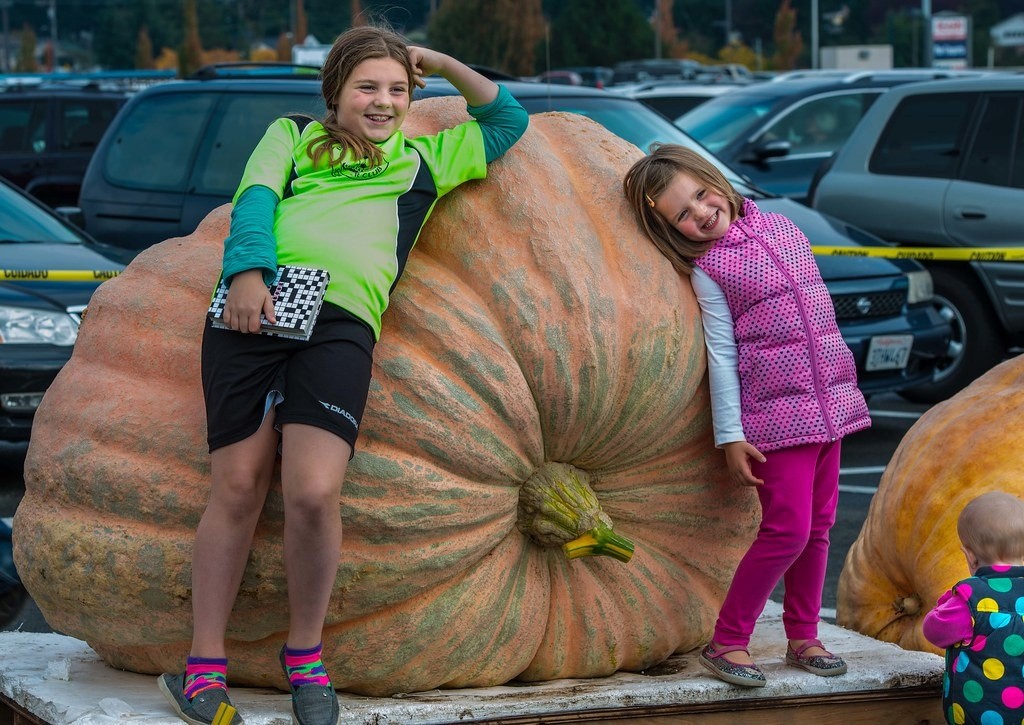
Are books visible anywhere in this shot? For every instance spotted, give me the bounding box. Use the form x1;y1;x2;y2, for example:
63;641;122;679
206;265;330;340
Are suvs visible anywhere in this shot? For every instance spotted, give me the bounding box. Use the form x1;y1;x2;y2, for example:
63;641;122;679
809;68;1024;400
647;67;983;204
81;56;951;404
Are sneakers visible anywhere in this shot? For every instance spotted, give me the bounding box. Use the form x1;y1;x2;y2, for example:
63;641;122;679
281;647;340;725
158;662;244;725
698;644;767;688
786;638;849;678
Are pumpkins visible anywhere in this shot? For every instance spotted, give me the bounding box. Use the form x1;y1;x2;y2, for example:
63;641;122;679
834;357;1024;657
12;111;762;696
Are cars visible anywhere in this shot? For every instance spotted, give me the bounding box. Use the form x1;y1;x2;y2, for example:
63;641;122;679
0;178;139;472
0;82;138;208
535;57;776;146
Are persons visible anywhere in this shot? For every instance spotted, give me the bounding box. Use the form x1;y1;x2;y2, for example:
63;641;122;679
156;26;528;725
923;491;1024;725
625;143;872;686
